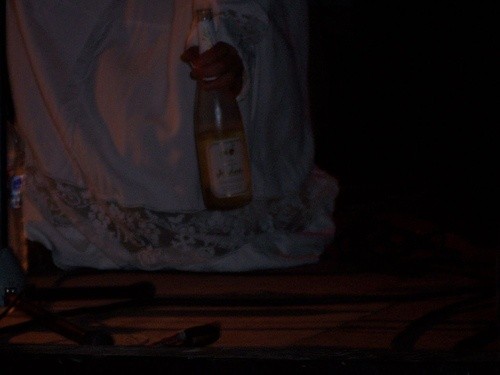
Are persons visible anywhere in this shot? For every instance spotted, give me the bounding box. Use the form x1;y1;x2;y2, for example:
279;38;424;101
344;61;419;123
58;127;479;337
3;0;340;272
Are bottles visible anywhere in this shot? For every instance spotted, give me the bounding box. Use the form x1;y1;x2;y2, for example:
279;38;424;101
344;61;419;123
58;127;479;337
191;7;254;212
